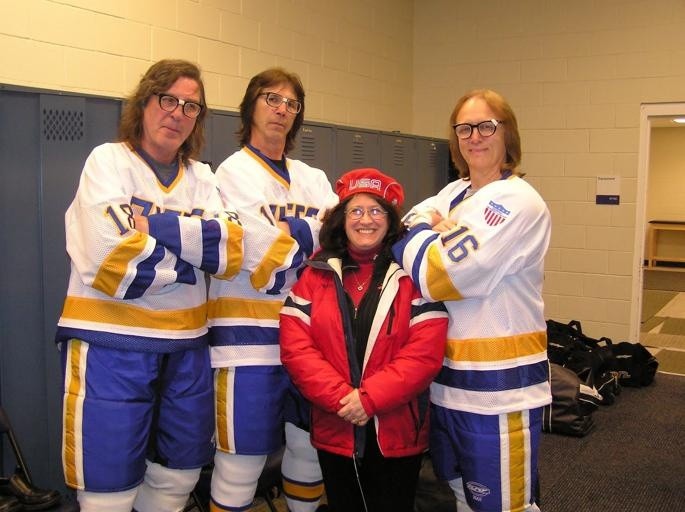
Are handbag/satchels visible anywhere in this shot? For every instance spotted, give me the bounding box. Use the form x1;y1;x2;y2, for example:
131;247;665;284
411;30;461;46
540;319;659;437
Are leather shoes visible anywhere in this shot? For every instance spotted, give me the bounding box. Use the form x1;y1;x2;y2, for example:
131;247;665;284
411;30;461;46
0;472;62;506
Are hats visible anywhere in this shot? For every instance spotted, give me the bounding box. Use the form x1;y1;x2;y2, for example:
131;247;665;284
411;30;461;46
336;168;405;215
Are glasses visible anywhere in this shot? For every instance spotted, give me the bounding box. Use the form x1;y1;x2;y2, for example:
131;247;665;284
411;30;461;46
344;207;388;220
452;118;504;139
258;92;301;115
152;91;204;120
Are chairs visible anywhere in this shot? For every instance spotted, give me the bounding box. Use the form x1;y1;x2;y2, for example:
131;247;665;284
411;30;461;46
1;404;64;512
180;381;304;512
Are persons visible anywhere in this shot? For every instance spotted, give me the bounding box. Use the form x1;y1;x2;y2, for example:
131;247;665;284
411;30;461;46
392;90;552;512
279;192;449;512
207;67;339;512
52;58;245;512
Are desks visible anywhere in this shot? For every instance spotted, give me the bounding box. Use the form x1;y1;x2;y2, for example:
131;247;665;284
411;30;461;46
645;218;685;274
